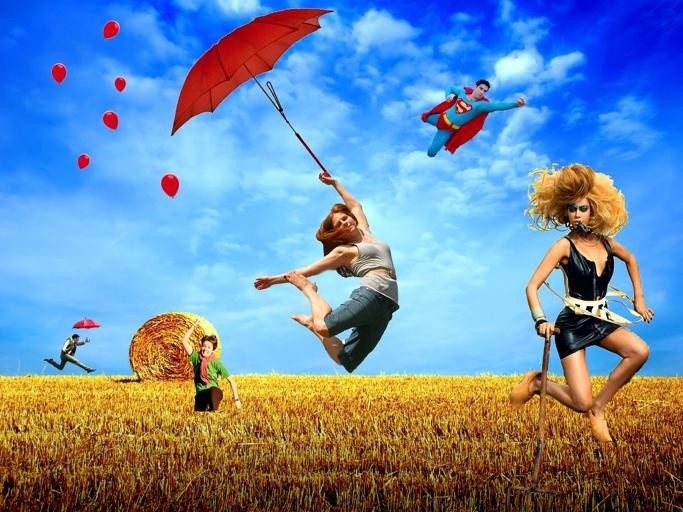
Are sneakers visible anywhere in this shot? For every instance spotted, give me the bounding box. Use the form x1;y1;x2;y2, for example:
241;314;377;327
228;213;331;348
44;358;53;361
87;368;96;373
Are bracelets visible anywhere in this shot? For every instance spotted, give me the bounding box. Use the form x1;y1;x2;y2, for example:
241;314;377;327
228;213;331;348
531;309;546;321
234;398;240;401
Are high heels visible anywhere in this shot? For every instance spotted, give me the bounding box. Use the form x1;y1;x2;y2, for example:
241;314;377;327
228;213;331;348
590;418;613;443
509;370;542;406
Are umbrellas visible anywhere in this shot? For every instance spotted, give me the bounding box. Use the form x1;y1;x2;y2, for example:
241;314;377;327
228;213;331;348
171;9;335;177
72;316;102;342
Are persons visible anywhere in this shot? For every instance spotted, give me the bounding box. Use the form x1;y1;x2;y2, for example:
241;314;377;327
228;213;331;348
420;80;526;157
523;163;655;445
254;170;400;375
43;334;96;373
182;318;241;411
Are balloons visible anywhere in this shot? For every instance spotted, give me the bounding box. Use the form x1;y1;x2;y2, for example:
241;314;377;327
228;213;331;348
114;76;126;92
160;173;179;199
103;21;120;38
102;111;118;130
78;154;90;169
51;63;68;84
